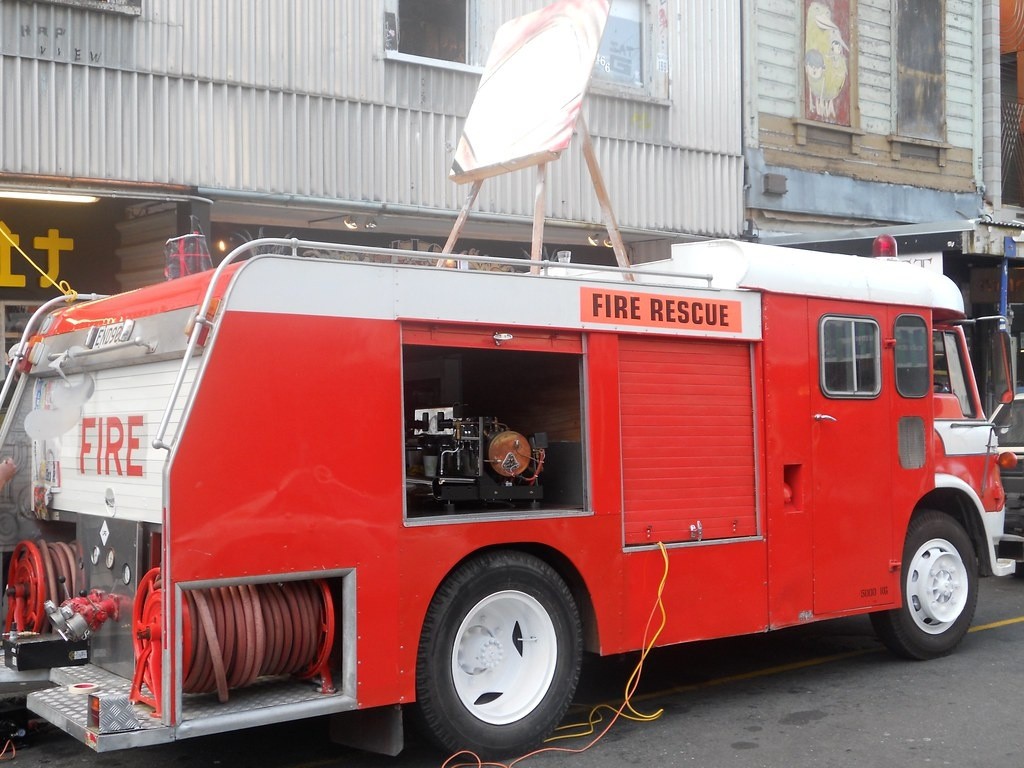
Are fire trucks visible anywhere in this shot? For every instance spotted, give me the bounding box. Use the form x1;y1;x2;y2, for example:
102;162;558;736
0;231;1017;768
